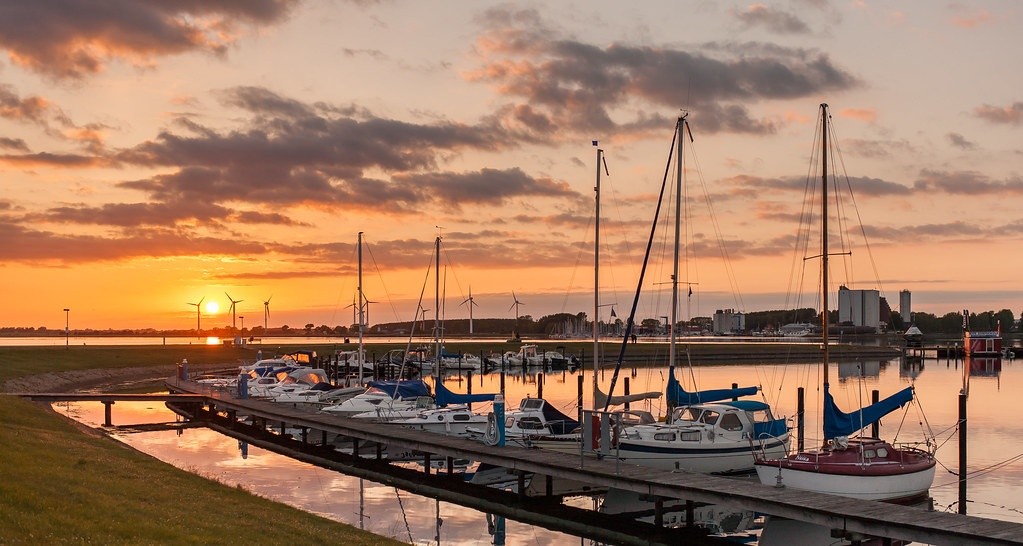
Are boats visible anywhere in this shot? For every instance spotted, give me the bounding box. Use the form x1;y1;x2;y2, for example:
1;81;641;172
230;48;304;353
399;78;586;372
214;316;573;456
233;414;913;546
198;222;579;447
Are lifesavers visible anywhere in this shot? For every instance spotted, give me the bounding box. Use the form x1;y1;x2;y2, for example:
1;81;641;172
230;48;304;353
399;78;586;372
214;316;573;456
599;417;619;447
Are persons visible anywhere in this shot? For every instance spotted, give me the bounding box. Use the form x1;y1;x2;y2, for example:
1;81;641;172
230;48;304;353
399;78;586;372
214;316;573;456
250;335;254;344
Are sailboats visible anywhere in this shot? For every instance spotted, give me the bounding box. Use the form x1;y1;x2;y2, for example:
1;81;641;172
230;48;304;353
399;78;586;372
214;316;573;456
594;108;794;478
509;140;664;447
743;102;938;503
